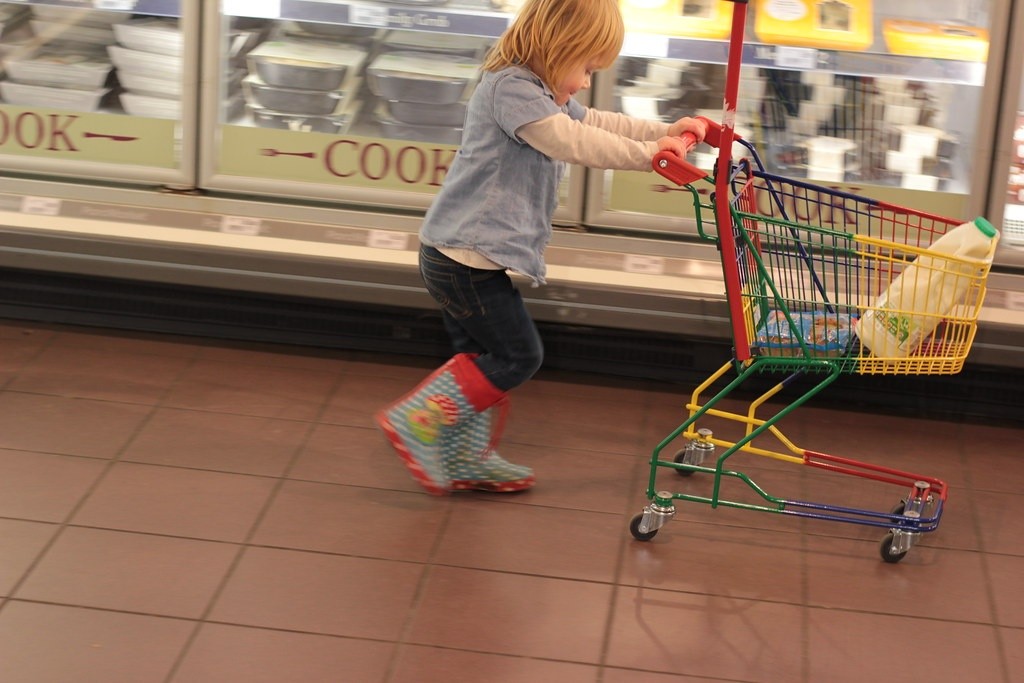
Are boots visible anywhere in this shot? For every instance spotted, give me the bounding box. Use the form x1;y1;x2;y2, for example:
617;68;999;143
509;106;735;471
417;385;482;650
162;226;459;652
375;349;510;498
437;406;536;492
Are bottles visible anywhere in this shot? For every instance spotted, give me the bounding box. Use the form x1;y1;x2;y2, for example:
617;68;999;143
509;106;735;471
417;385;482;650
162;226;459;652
855;217;996;363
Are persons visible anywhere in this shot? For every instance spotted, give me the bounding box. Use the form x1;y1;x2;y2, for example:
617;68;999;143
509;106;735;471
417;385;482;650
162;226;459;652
374;0;707;496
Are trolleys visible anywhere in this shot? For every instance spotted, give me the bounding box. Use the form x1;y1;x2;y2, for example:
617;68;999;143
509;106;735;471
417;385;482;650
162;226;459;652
630;116;1000;563
